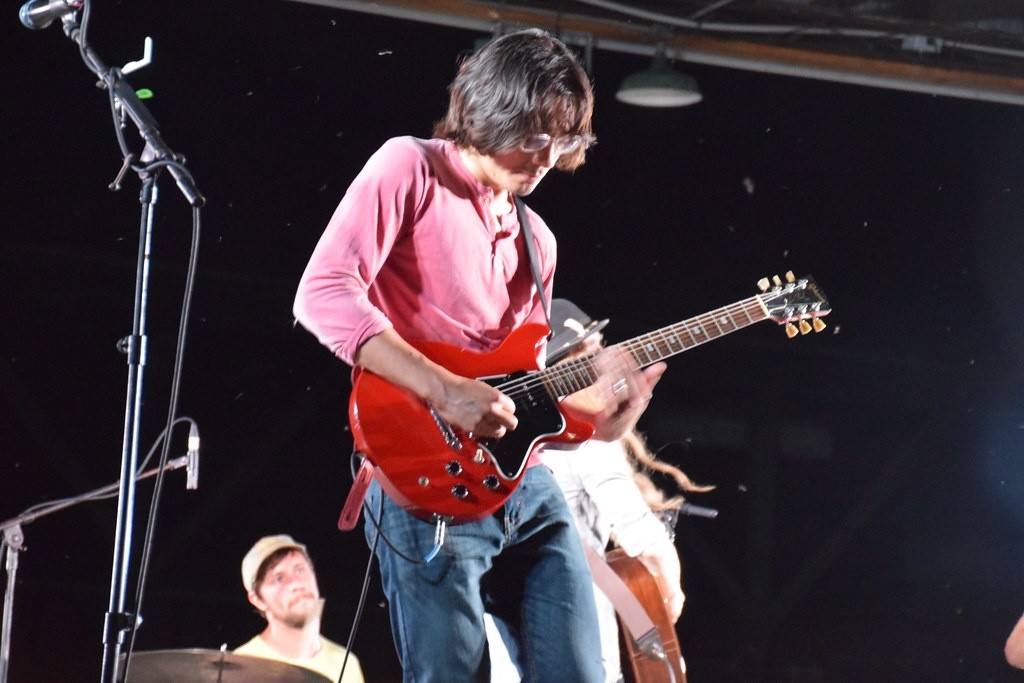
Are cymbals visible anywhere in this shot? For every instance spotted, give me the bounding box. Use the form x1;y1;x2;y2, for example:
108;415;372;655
117;648;339;683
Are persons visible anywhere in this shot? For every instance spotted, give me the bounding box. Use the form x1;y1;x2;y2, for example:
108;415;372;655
487;300;684;680
287;24;668;683
1003;612;1023;669
228;535;366;683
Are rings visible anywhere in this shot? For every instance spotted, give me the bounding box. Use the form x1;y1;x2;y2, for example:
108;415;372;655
611;377;628;394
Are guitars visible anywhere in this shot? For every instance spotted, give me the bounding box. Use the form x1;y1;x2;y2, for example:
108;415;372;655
349;270;831;522
604;485;718;683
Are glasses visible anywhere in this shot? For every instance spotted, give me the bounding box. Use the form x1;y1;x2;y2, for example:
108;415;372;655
521;133;582;154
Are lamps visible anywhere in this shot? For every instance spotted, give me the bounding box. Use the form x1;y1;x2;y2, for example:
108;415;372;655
615;50;700;107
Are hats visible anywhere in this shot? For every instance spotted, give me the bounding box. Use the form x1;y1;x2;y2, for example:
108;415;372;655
547;299;609;365
240;534;307;592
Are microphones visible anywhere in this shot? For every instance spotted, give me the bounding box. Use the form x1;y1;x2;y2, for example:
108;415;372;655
187;423;200;491
19;0;86;31
